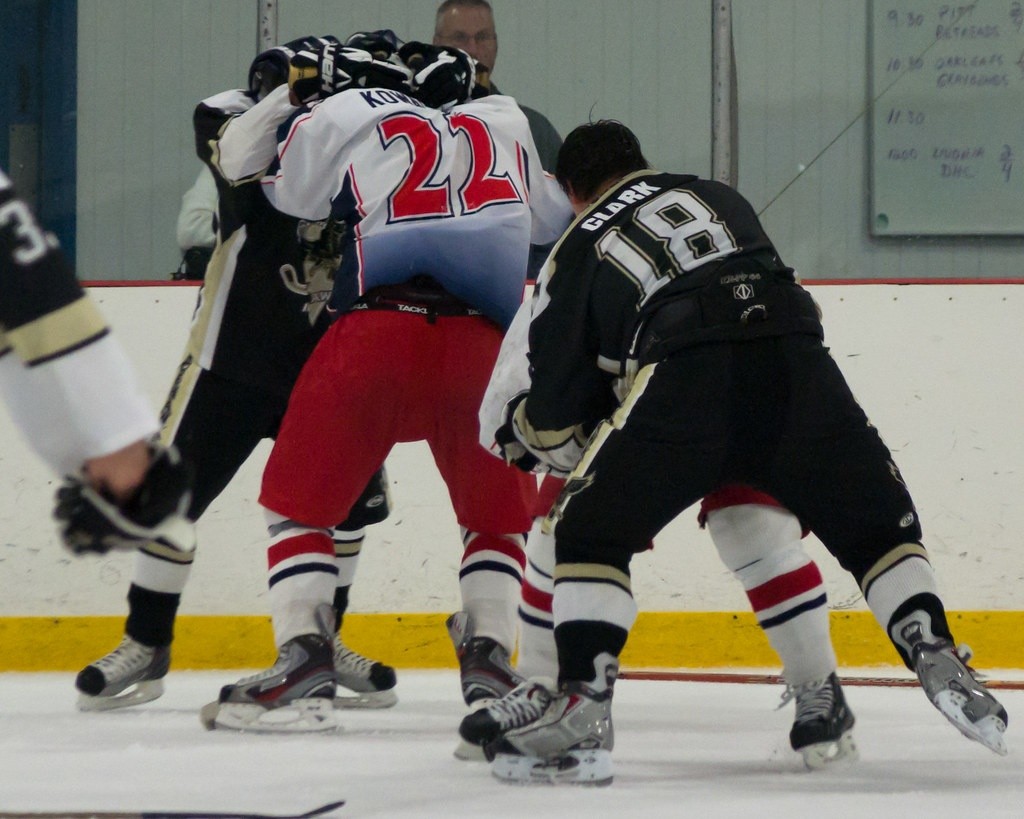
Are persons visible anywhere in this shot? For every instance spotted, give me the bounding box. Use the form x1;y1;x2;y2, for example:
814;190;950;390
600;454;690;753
1;168;192;554
201;41;574;734
428;2;563;175
457;207;865;763
74;35;480;709
487;121;1008;786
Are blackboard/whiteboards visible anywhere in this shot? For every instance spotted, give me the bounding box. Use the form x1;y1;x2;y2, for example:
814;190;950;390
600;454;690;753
863;0;1024;240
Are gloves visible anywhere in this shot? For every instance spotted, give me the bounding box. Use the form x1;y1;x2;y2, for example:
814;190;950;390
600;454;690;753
61;442;193;556
496;391;588;473
398;41;488;110
345;30;398;62
294;42;416;103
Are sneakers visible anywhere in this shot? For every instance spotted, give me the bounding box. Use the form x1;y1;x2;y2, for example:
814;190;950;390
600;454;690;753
336;641;398;711
791;672;860;768
461;639;524;702
74;640;170;710
893;641;1010;754
457;677;552;772
492;653;622;784
202;634;337;732
